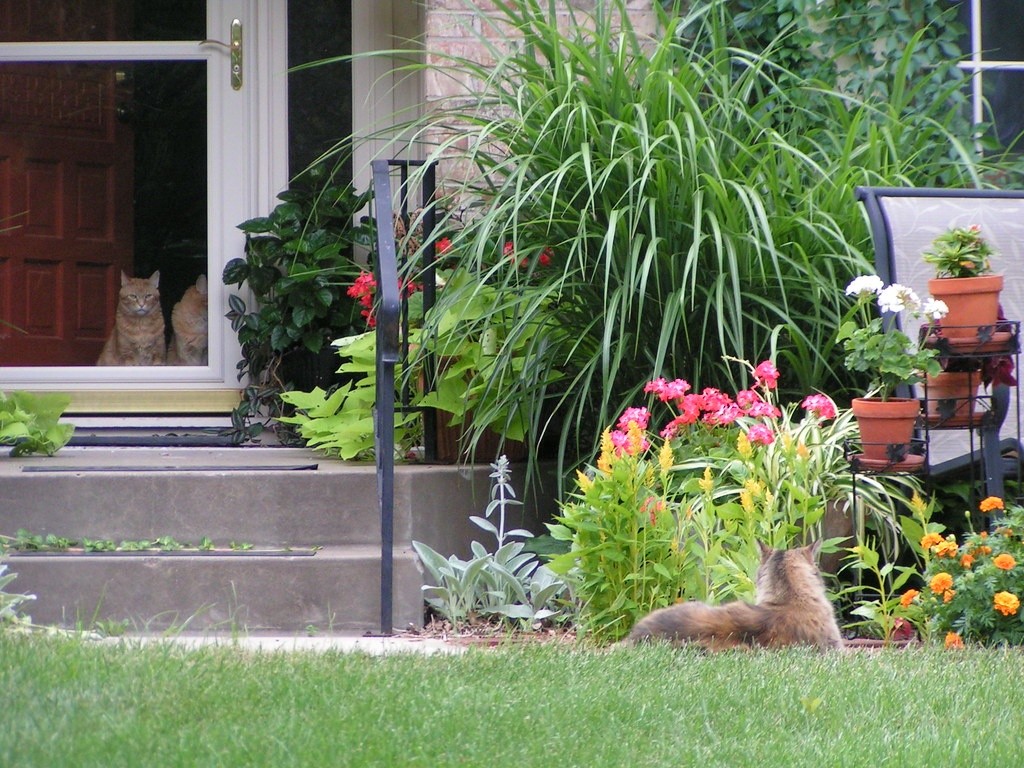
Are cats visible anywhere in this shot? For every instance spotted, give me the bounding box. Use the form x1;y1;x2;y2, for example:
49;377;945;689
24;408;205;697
166;273;208;366
96;270;167;367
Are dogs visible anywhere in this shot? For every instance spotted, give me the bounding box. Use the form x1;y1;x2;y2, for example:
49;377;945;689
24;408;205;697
608;534;846;658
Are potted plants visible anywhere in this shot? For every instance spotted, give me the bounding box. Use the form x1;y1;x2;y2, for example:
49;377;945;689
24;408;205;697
847;396;934;474
923;224;1004;338
919;363;982;418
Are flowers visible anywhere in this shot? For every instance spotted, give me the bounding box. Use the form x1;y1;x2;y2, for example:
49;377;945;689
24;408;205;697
833;271;951;400
